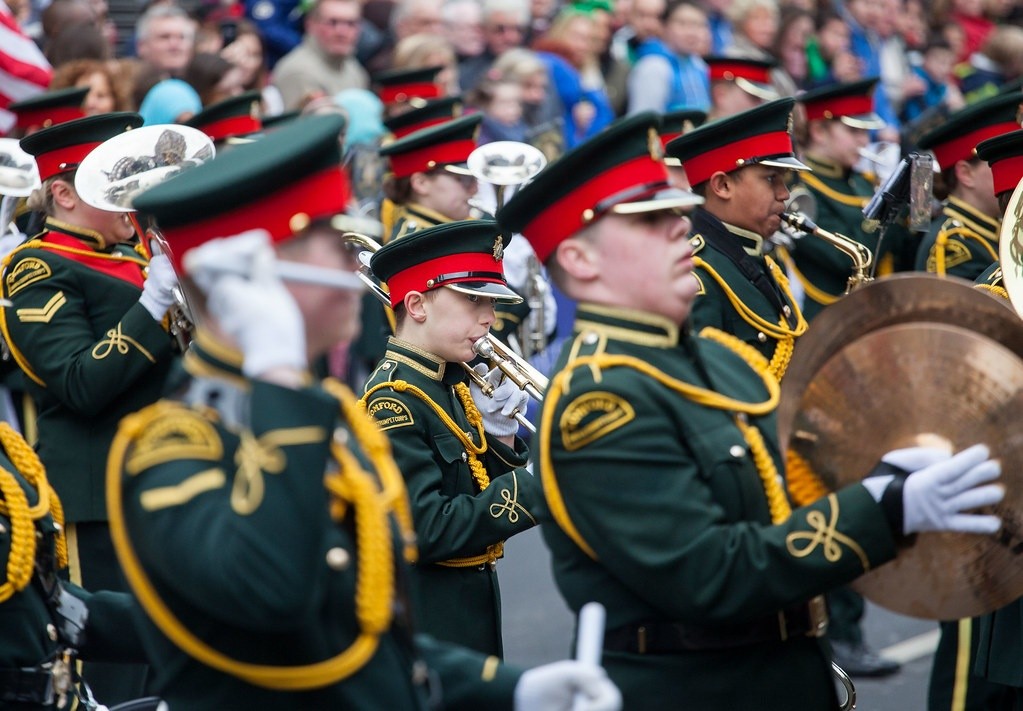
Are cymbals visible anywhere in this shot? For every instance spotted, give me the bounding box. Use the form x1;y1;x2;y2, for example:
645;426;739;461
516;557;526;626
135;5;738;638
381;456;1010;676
777;272;1023;464
784;322;1023;621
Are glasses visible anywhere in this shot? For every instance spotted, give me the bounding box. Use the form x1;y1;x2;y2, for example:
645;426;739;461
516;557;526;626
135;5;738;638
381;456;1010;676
329;17;356;27
494;25;526;36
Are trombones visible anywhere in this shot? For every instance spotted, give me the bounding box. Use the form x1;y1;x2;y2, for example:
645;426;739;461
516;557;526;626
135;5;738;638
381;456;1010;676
341;231;549;436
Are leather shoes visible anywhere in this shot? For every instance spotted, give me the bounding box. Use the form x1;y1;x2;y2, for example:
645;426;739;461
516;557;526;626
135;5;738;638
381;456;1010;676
830;641;900;678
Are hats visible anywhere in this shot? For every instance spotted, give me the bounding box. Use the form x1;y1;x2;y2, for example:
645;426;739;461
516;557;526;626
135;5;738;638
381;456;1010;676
181;90;263;141
798;78;885;129
977;131;1023;197
915;92;1023;170
379;113;482;179
664;97;813;187
496;111;705;264
384;95;459;141
372;66;443;105
702;55;781;101
8;88;94;128
262;110;299;130
654;110;708;148
137;114;354;278
370;221;523;305
20;112;144;183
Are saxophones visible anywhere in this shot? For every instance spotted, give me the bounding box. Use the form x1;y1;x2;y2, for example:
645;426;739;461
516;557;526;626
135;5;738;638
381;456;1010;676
778;211;875;297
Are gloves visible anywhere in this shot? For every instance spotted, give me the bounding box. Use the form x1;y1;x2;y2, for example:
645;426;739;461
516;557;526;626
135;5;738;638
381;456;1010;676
470;363;530;437
186;228;309;374
528;296;557;335
882;444;1004;535
514;602;622;710
138;254;181;321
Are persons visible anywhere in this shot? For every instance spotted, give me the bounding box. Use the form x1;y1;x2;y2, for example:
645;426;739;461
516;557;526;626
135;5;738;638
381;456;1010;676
497;111;1006;711
0;0;1023;711
344;220;555;665
103;115;625;711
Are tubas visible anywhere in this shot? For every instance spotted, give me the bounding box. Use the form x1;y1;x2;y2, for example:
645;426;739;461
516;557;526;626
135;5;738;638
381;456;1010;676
0;137;43;240
466;141;559;357
74;123;217;352
1000;175;1022;320
